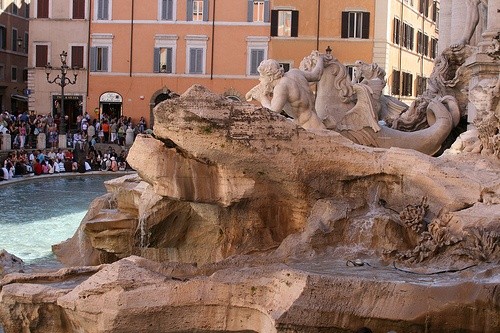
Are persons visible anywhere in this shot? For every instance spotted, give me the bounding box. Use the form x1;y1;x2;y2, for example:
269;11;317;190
0;105;150;180
254;57;326;131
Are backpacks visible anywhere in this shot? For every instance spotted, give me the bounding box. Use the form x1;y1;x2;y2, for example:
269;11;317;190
8;161;16;177
101;159;108;170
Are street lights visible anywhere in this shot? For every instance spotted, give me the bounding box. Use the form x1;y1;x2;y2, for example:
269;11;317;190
45;50;80;134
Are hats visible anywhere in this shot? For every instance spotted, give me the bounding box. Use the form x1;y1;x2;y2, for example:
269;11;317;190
110;156;115;161
104;153;107;157
